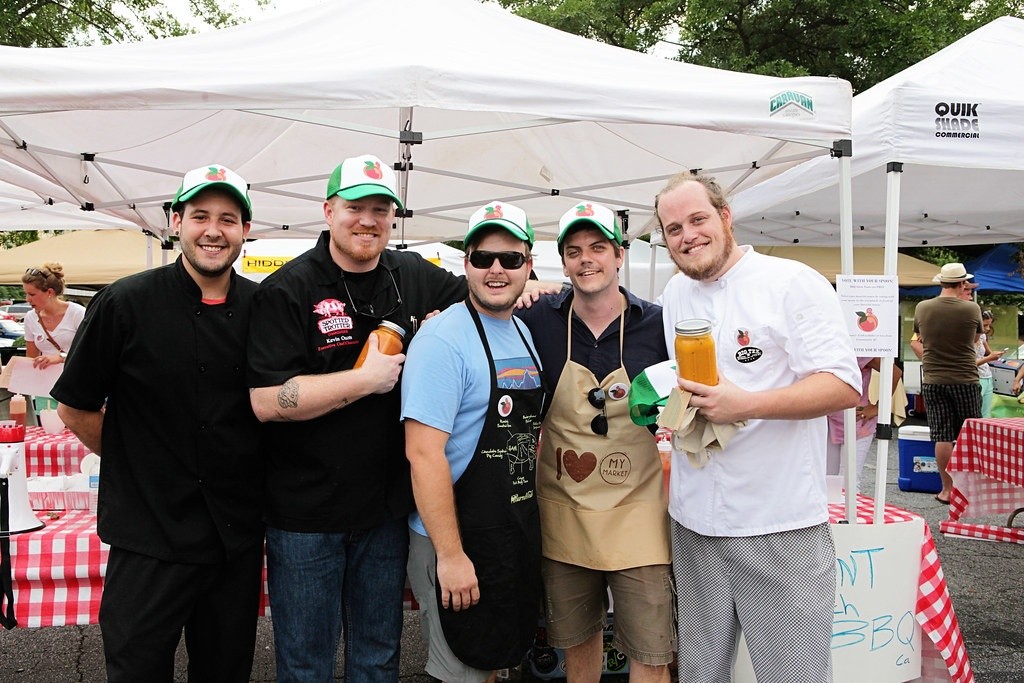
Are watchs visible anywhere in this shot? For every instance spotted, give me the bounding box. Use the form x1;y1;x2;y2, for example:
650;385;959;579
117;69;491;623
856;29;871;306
59;353;67;363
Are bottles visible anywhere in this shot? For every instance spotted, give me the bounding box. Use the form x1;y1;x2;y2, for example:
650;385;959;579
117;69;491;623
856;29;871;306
10;394;26;435
675;319;717;388
88;460;99;513
352;321;405;370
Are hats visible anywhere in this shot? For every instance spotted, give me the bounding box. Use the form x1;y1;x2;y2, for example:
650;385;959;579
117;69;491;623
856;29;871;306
557;200;622;245
170;164;253;222
628;360;678;426
932;263;974;283
963;281;979;289
463;200;534;252
326;154;403;210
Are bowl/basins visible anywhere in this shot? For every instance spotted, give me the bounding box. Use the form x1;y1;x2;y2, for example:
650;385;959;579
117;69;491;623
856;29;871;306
40;410;65;434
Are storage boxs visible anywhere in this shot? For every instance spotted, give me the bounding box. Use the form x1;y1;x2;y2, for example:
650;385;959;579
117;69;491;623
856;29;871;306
524;614;631;683
66;475;89;509
25;475;76;509
898;425;957;494
988;359;1024;397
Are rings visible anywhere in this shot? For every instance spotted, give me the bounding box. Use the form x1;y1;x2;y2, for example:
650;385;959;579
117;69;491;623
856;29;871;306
39;358;42;362
39;356;42;358
861;414;865;418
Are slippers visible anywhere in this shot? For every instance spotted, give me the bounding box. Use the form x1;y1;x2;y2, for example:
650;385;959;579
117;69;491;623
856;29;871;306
935;494;950;505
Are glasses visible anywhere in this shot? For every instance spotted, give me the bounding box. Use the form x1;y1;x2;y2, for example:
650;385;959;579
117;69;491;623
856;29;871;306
25;268;48;279
469;249;528;269
343;263;403;319
587;388;608;437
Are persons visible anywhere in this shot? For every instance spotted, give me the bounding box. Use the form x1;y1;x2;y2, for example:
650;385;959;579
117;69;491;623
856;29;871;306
1012;365;1024;395
249;156;863;683
49;164;259;683
21;262;86;371
911;262;1008;505
826;282;902;494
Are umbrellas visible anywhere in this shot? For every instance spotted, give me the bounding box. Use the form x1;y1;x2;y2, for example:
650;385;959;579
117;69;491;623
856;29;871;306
0;229;468;285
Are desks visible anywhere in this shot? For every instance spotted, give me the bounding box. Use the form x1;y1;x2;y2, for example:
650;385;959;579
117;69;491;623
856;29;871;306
0;418;420;627
731;488;975;683
945;417;1024;527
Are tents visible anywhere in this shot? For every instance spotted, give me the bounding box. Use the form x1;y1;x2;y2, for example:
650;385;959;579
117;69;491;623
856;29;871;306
648;17;1024;514
531;238;1024;303
0;0;857;524
